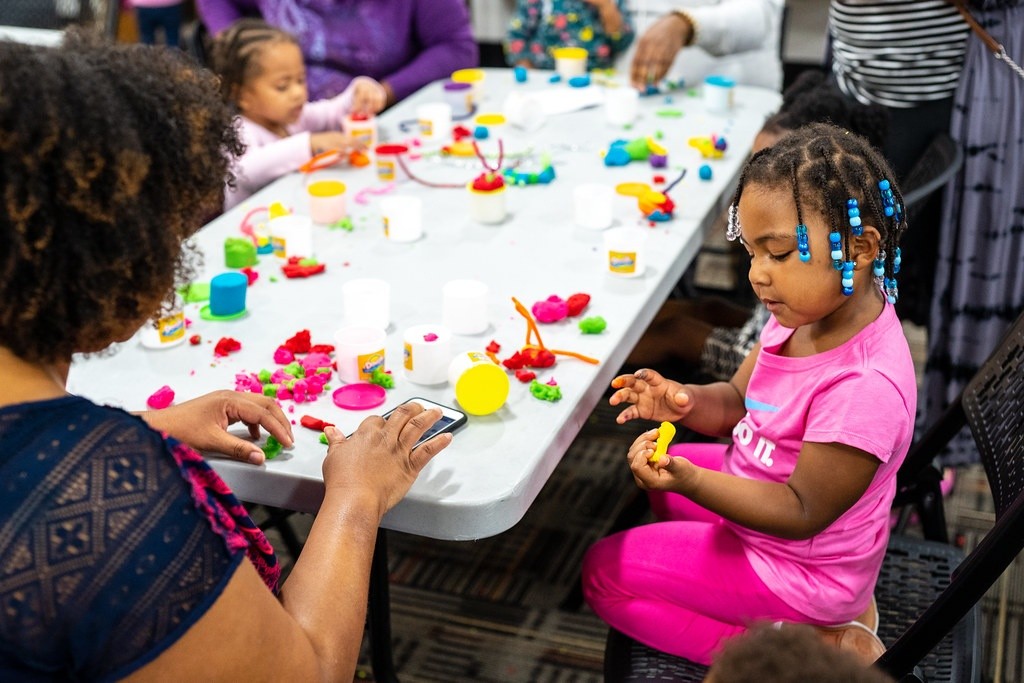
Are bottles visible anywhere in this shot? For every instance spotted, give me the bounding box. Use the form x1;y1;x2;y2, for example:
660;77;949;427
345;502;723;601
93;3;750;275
447;350;510;415
606;225;647;277
443;68;485;121
139;294;187;349
309;181;346;225
553;46;588;83
381;191;423;242
466;180;507;224
269;213;313;263
344;112;378;151
332;324;388;383
402;324;452;384
374;143;410;184
705;75;734;111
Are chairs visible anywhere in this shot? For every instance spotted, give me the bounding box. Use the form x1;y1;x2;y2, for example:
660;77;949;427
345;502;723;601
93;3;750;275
602;304;1024;683
900;132;965;211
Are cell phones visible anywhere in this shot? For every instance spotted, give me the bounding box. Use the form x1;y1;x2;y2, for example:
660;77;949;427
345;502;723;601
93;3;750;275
344;397;467;454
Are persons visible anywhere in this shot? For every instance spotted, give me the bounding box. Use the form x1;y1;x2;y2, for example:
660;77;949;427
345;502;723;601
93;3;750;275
214;20;386;214
623;73;889;382
503;0;776;93
584;122;915;670
129;0;479;120
0;39;458;683
703;620;894;683
783;0;1024;528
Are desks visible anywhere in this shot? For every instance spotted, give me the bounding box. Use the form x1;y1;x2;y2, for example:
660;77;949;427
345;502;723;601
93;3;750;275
65;66;785;683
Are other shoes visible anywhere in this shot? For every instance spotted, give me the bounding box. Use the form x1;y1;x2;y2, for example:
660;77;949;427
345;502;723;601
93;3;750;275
772;594;887;667
